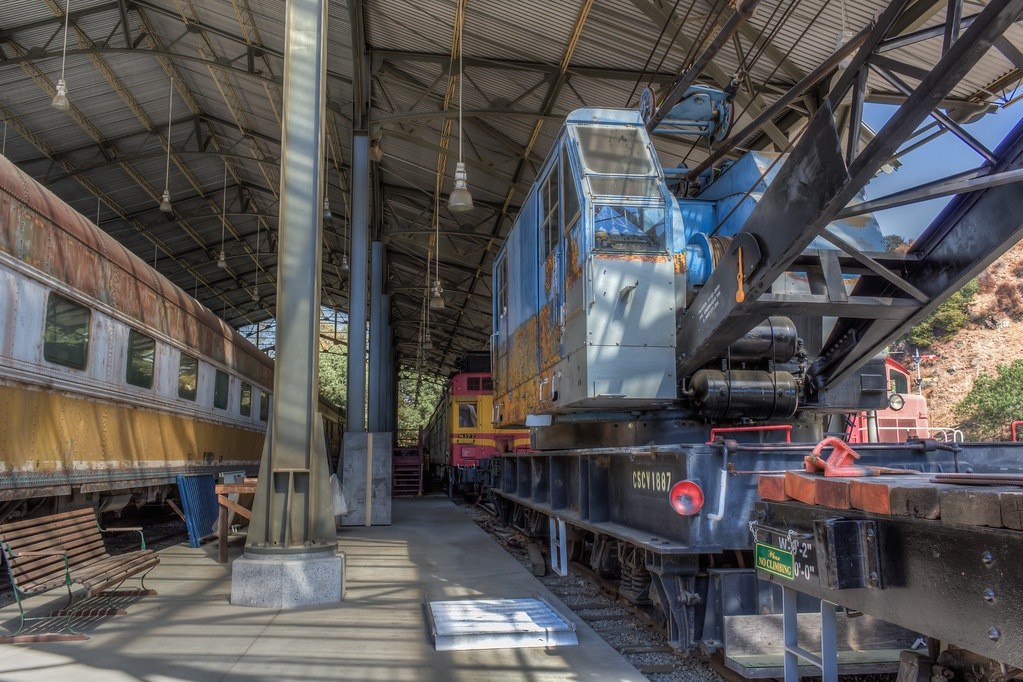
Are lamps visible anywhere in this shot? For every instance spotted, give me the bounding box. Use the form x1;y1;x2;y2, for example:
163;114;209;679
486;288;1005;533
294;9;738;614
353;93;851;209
422;252;433;349
51;0;69;113
342;207;349;271
158;77;171;211
322;135;331;218
431;172;446;309
218;161;227;266
446;5;475;213
836;0;860;69
251;216;260;301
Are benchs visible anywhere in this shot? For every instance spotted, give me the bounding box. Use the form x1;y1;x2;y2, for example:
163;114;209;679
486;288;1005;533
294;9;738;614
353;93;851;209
0;505;160;645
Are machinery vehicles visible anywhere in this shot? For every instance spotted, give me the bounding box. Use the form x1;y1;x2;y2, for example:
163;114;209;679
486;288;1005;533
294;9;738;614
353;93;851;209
486;0;1023;681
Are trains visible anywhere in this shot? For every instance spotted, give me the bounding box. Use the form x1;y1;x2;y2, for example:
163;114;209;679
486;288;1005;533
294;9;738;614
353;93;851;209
424;354;532;506
2;159;349;542
838;350;936;448
